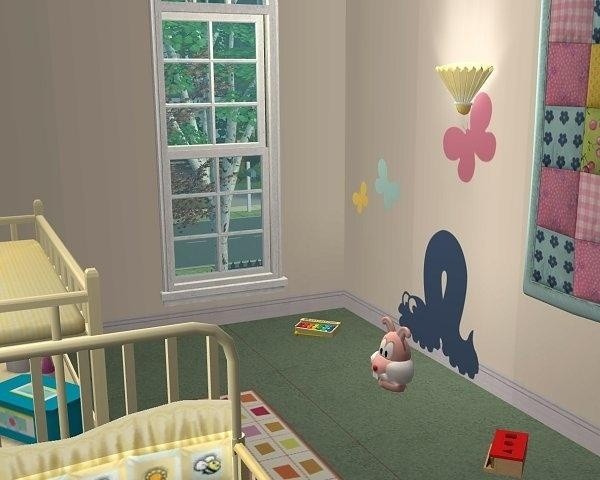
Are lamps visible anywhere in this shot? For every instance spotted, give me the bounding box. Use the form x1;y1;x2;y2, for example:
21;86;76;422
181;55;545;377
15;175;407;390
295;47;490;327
434;61;494;117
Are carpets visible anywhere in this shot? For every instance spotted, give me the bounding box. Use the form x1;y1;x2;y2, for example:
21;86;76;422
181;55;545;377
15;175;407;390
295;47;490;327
220;388;346;480
